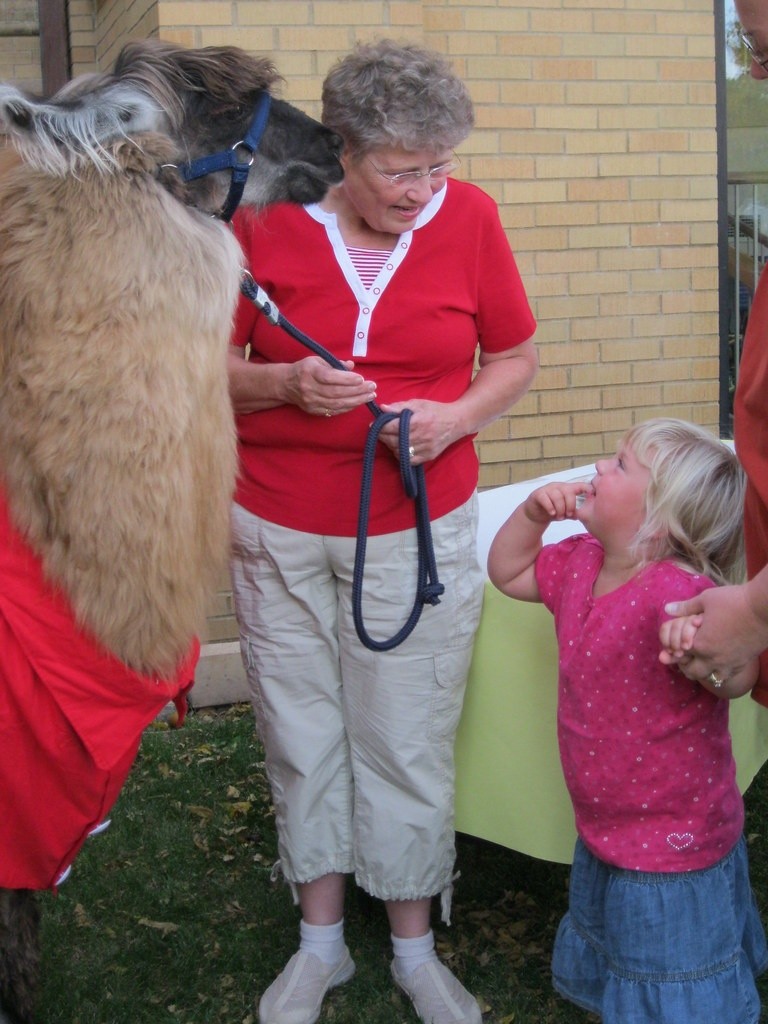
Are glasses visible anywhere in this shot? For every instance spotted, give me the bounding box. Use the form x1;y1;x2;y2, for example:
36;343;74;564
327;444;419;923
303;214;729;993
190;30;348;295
364;149;463;186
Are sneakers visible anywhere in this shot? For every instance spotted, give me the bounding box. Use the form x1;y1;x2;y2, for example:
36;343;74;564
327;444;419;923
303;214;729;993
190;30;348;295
391;955;483;1024
259;946;356;1024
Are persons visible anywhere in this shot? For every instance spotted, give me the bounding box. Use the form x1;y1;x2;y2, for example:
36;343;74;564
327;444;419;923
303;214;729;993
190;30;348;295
483;415;767;1024
656;1;767;706
221;37;537;1024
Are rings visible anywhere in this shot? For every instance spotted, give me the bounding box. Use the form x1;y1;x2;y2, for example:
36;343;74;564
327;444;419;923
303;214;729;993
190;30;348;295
711;672;723;688
407;446;416;460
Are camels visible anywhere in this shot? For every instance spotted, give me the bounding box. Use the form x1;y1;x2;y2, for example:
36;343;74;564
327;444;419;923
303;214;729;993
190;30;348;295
0;44;343;895
